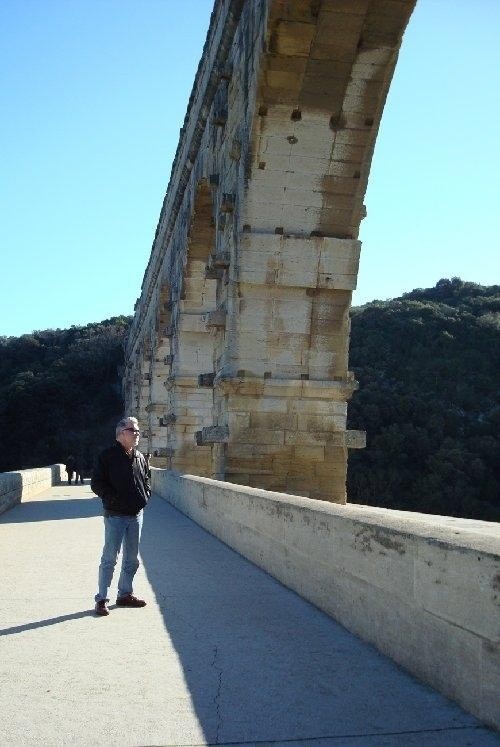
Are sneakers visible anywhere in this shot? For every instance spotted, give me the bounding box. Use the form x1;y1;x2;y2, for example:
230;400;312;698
95;602;109;615
67;479;84;483
116;595;147;607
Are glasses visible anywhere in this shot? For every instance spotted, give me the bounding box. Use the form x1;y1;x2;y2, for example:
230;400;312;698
122;428;139;432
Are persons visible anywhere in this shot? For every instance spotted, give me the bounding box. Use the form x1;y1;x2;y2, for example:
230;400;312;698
73;452;87;486
65;455;76;486
146;453;152;470
144;454;147;460
90;415;152;616
